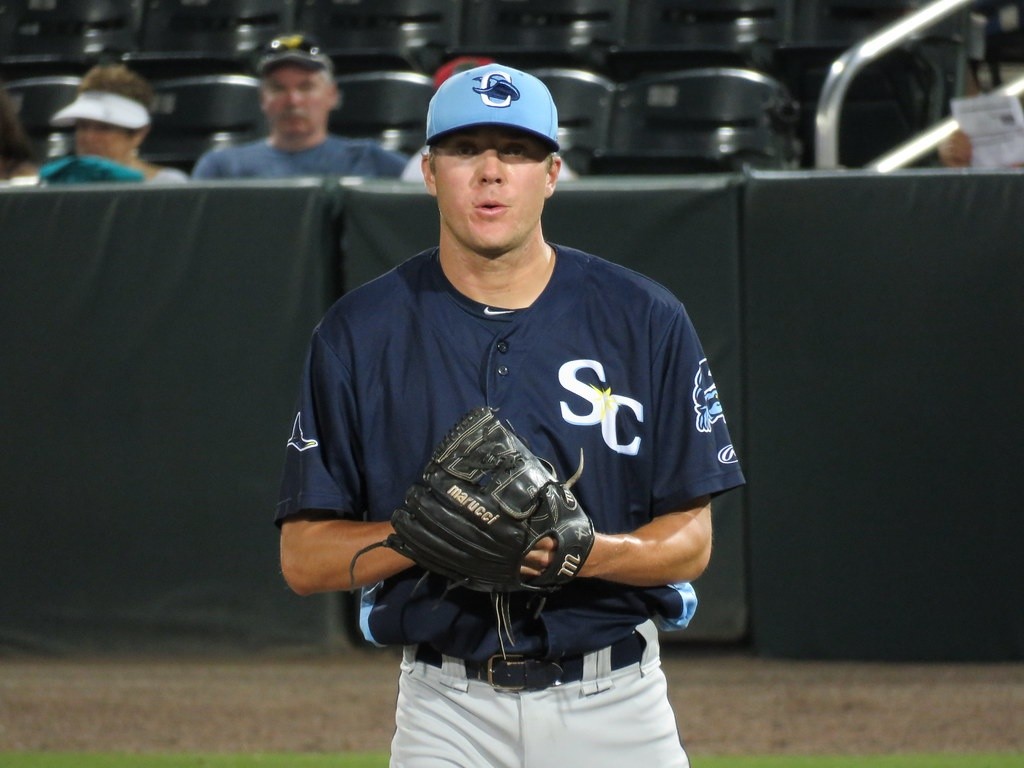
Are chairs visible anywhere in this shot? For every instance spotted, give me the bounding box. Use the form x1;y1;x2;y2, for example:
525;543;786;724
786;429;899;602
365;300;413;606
0;1;1023;176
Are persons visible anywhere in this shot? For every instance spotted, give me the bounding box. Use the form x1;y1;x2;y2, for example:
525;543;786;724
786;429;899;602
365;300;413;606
0;30;581;188
274;63;747;768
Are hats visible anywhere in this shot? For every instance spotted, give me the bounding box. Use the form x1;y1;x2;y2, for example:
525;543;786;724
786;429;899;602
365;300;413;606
248;33;330;74
425;63;560;153
49;91;152;130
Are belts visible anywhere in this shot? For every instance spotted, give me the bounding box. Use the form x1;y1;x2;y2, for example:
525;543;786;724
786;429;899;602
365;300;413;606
415;632;646;693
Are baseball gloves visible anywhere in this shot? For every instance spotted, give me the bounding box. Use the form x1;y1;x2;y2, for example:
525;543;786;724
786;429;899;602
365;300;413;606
379;405;597;599
